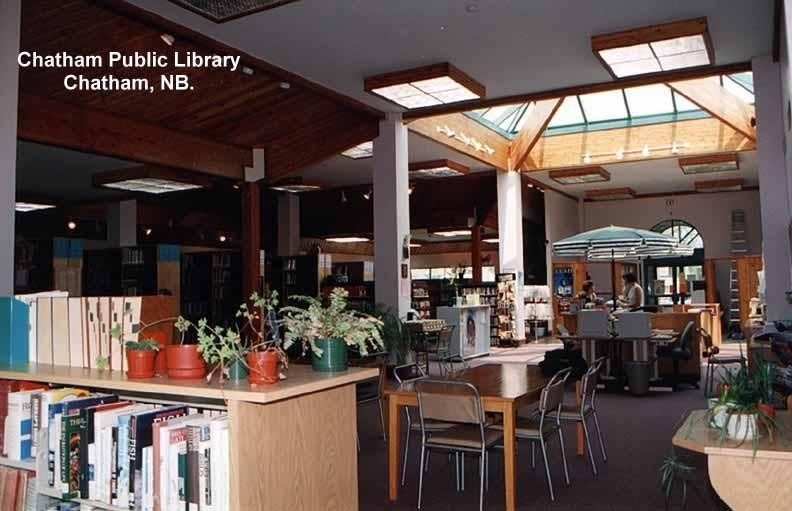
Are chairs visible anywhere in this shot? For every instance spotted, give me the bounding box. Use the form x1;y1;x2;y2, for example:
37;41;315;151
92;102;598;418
656;321;700;392
348;309;608;508
697;328;746;398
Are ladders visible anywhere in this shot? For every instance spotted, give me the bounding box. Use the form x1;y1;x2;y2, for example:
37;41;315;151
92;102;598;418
727;209;747;340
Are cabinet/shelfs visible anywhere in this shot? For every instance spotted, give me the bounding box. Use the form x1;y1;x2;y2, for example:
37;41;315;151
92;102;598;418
554;260;639;332
736;255;762;338
14;234;516;345
0;359;379;511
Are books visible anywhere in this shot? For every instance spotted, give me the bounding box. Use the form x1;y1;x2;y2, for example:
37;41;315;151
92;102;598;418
3;287;221;511
413;288;432;321
176;251;238;344
463;288;499;346
318;285;373;315
280;258;298;304
119;247;152;296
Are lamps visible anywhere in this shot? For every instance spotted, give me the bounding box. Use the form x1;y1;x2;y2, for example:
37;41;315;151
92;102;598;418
13;1;743;249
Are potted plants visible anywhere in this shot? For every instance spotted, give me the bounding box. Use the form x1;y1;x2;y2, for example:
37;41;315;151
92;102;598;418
96;287;389;387
708;348;790;464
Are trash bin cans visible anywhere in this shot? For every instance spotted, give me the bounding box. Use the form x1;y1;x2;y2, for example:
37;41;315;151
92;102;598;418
623;359;650;396
529;320;548;338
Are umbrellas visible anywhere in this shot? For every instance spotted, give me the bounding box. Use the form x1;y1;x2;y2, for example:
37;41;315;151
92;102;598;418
585;244;695;305
552;223;679;314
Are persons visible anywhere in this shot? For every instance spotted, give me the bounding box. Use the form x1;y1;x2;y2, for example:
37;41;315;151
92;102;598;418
574;279;600;310
615;272;645;314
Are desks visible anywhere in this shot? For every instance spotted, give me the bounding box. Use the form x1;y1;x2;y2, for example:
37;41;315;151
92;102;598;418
555;328;679;393
672;408;792;511
